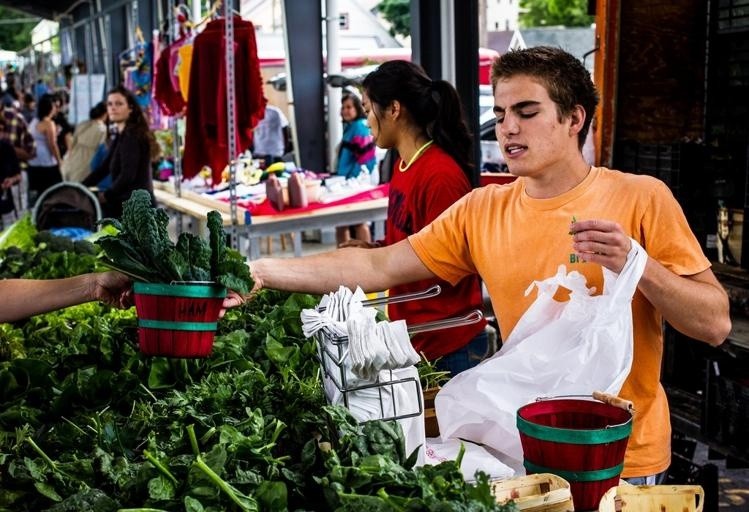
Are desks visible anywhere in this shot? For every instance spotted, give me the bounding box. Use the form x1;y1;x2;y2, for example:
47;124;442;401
154;180;390;261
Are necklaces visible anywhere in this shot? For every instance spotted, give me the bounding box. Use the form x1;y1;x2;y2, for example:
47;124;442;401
398;139;433;173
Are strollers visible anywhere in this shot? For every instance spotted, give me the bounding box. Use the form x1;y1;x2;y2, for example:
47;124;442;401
28;180;104;242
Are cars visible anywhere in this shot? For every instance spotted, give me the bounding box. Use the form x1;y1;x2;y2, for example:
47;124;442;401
478;75;597;176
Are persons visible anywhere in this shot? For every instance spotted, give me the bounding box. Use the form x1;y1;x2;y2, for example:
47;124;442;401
250;107;286;184
0;270;133;324
338;59;490;389
336;94;377;243
0;62;158;224
218;45;733;486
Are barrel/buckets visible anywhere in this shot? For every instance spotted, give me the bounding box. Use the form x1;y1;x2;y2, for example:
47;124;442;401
128;280;230;360
514;390;640;512
